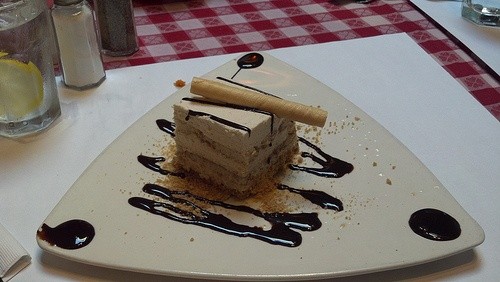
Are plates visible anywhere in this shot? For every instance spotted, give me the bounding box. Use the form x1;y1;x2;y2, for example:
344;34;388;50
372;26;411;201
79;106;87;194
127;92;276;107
36;51;486;282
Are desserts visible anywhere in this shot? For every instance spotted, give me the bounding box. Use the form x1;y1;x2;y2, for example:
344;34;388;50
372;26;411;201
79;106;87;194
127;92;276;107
171;76;330;200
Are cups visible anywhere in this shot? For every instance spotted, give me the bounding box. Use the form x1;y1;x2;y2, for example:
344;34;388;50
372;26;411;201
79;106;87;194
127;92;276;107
461;1;499;29
0;0;62;138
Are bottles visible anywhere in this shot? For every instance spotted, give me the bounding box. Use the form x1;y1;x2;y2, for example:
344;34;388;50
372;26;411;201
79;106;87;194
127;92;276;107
50;0;106;90
93;0;140;55
